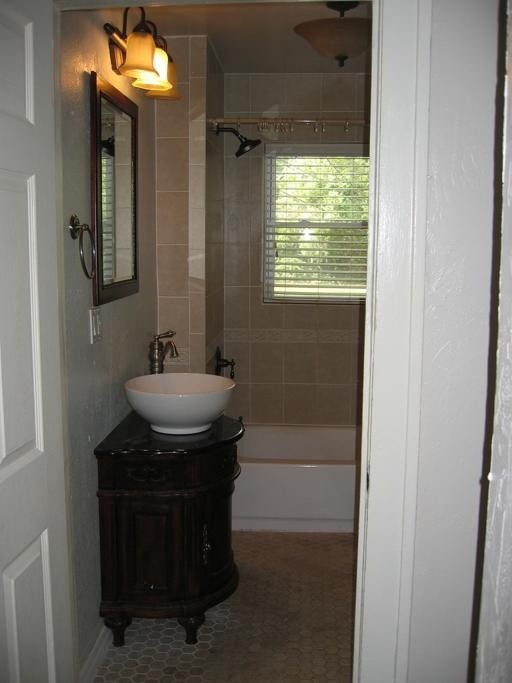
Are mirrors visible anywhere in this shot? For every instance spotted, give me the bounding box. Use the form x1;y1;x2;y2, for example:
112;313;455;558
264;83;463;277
87;65;142;304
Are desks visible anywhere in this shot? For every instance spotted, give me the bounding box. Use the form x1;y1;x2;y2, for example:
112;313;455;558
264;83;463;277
95;408;246;649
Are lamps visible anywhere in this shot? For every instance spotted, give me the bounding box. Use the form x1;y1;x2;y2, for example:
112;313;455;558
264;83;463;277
292;0;371;68
104;7;178;101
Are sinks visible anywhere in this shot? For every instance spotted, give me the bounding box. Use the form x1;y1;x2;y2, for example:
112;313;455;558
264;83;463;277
124;373;236;435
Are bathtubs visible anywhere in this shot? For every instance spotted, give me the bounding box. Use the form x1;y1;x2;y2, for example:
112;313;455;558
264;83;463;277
229;426;362;532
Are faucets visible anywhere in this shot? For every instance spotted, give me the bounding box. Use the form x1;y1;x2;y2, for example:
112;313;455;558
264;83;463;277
149;329;178;374
